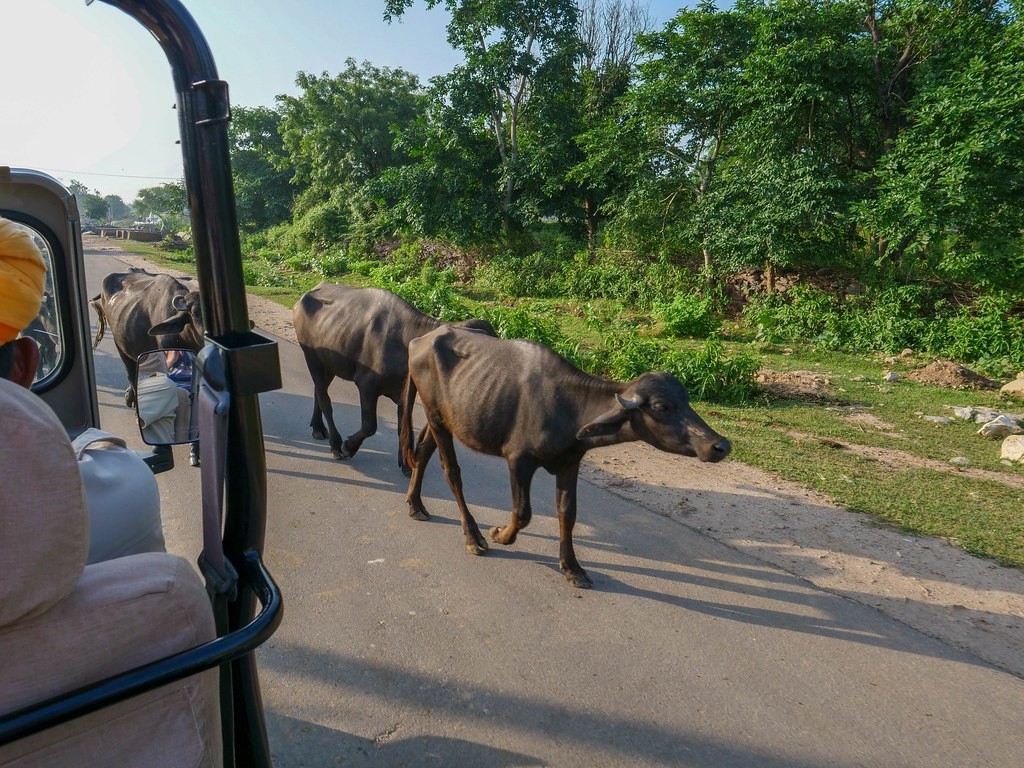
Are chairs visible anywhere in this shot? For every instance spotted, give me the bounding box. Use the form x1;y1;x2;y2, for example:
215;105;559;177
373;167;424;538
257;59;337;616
174;387;191;442
1;371;226;768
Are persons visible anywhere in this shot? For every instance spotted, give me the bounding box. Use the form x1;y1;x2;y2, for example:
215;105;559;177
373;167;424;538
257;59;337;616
137;350;193;444
0;218;167;565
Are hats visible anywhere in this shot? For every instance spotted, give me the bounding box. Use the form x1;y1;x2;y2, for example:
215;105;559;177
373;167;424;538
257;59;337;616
0;215;47;346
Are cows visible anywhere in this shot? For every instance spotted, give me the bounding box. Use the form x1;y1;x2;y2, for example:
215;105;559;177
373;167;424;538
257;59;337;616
292;281;733;589
90;266;255;466
